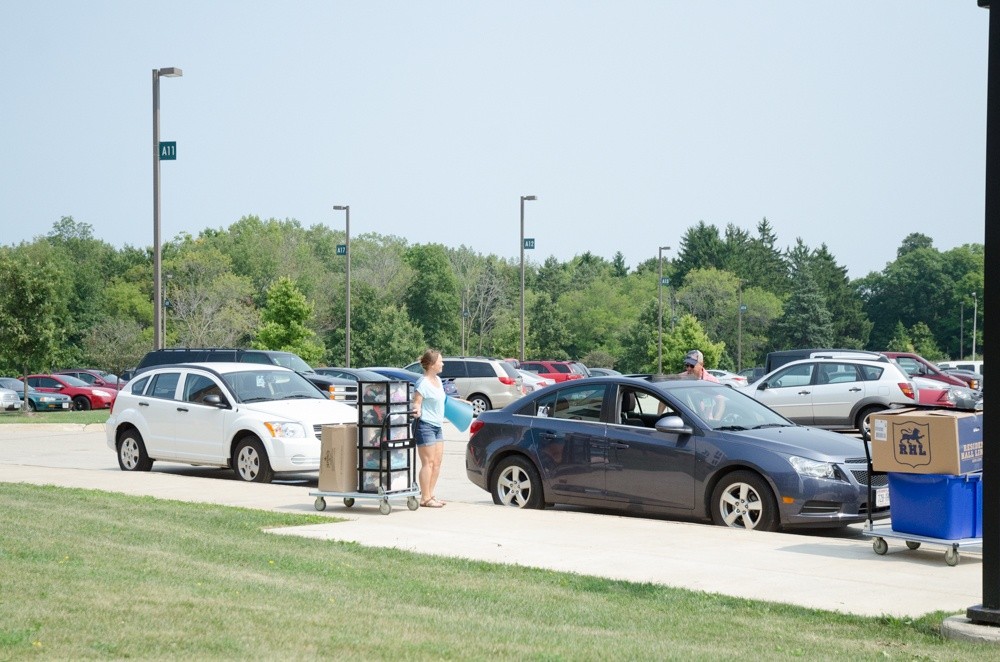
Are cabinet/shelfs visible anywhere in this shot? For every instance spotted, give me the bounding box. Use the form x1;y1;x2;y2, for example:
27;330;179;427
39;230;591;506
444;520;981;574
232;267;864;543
357;379;411;494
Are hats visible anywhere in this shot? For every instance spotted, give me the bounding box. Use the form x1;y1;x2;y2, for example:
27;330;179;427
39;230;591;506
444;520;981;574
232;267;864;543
683;350;704;366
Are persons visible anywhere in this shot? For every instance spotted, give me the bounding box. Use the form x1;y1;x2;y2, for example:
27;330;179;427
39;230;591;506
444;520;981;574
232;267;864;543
409;348;474;508
656;349;725;422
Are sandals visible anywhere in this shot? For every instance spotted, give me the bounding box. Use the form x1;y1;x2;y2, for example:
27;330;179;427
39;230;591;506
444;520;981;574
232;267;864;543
420;496;447;508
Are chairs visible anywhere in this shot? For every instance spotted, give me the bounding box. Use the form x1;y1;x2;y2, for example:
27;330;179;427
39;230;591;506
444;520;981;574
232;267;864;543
621;392;646;427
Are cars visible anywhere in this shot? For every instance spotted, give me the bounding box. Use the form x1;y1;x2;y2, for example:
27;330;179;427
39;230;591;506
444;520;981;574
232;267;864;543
498;358;766;393
515;368;556;397
364;365;460;402
400;357;526;417
897;357;983;392
18;374;121;412
0;377;73;412
0;388;24;412
118;367;136;381
879;352;967;408
467;374;891;537
58;368;128;391
312;368;417;422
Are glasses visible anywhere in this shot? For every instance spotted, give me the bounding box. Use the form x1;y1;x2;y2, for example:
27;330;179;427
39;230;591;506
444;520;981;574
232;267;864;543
685;364;694;368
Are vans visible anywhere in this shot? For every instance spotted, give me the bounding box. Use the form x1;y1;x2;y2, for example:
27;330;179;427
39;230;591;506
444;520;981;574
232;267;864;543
936;360;984;377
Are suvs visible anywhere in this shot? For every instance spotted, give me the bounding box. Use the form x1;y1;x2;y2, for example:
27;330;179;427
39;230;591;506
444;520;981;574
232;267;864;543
104;359;361;485
766;349;882;374
134;346;361;407
739;350;921;439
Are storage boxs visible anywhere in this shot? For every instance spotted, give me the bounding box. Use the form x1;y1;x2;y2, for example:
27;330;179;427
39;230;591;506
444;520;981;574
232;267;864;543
887;472;983;540
318;424;358;492
869;407;983;477
